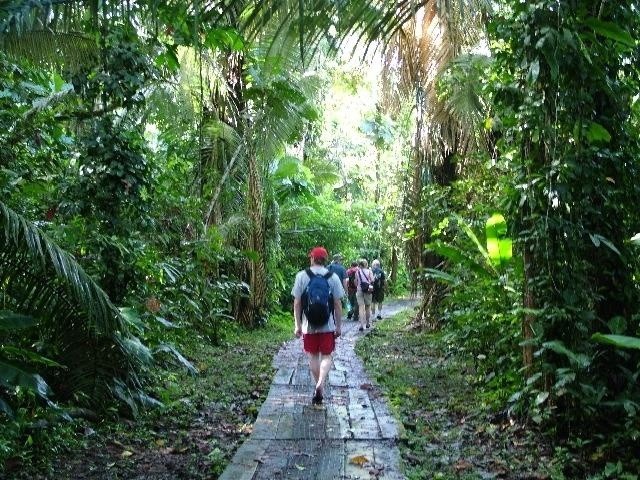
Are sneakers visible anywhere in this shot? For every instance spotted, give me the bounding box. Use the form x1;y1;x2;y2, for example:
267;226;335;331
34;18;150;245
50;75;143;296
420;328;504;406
312;388;323;402
346;314;382;331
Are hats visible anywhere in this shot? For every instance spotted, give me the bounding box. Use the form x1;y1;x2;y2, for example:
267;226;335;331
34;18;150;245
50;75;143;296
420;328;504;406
308;247;327;258
333;254;344;261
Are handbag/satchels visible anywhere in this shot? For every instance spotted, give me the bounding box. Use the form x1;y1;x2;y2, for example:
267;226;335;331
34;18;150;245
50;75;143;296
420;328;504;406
361;282;375;293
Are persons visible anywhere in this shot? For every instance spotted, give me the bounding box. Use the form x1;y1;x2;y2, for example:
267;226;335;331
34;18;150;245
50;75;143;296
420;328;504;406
370;258;386;322
346;260;360;322
291;246;348;404
327;253;348;325
354;258;376;332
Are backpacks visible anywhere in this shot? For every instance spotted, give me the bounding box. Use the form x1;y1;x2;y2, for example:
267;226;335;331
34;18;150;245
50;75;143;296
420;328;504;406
303;268;334;330
373;273;381;289
349;271;357;289
